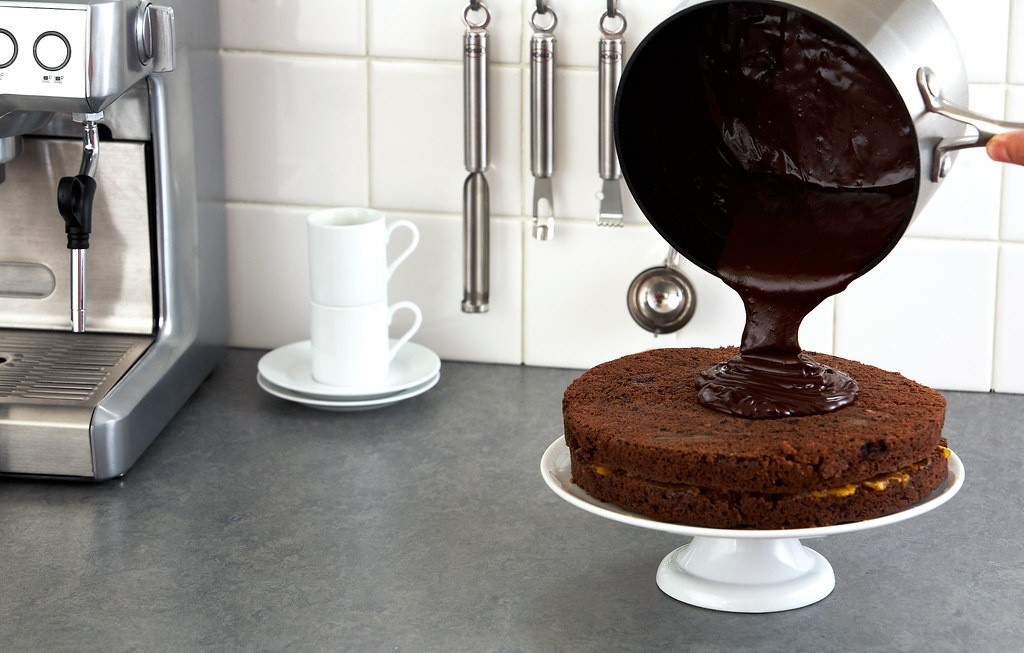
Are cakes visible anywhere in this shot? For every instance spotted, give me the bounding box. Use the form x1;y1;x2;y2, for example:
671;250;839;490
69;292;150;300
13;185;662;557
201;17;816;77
562;346;949;531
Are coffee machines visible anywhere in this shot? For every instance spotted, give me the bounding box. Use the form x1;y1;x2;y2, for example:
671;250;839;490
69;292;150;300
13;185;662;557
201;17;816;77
0;0;228;480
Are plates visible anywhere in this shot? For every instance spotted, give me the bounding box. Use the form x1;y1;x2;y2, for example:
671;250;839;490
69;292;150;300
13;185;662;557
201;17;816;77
258;340;441;401
541;434;965;614
257;371;441;411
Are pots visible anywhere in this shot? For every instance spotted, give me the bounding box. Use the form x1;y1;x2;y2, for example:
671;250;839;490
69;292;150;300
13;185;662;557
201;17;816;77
614;0;1024;289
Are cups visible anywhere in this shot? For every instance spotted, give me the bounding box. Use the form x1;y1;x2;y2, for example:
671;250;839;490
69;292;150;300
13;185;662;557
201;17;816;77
311;298;422;386
307;207;419;308
